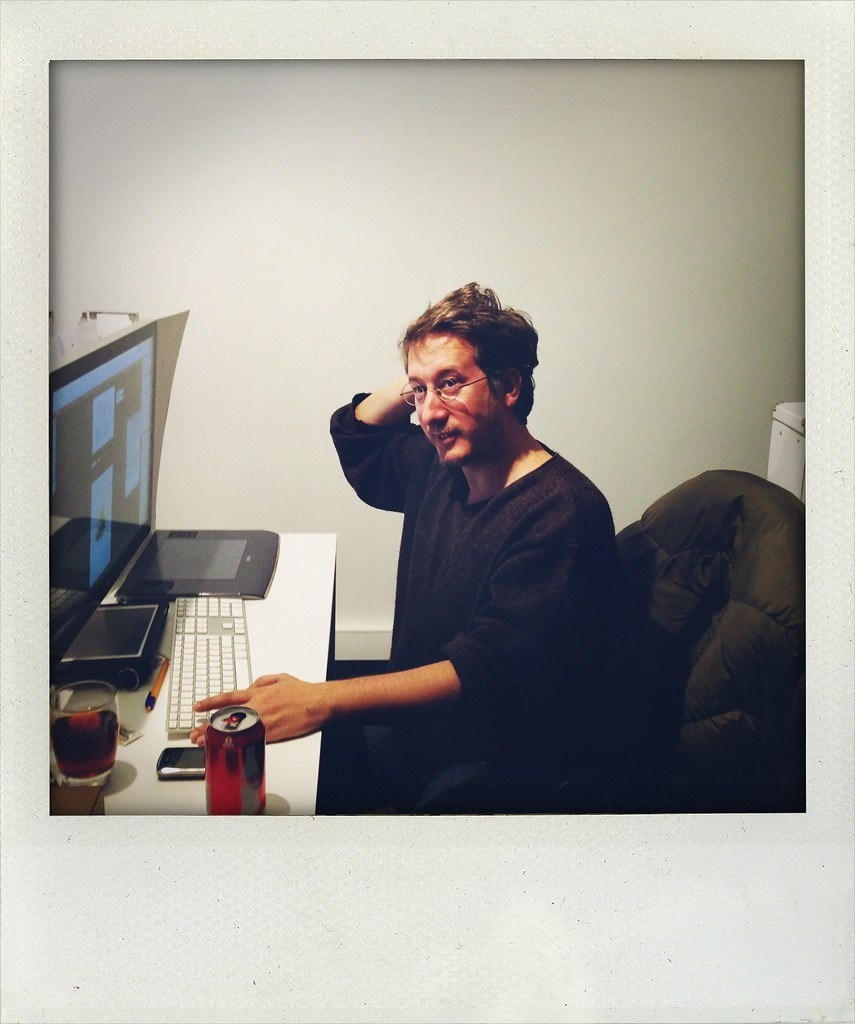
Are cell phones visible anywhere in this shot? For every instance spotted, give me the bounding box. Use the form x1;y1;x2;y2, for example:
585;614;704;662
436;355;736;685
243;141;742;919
156;748;206;779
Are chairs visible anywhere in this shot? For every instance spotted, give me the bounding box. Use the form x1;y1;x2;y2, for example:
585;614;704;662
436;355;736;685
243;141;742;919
589;466;806;817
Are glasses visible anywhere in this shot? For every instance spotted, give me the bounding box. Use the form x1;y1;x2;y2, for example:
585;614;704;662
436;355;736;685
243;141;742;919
399;371;502;406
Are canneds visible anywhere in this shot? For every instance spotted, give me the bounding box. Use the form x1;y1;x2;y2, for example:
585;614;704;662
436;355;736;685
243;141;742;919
202;705;267;815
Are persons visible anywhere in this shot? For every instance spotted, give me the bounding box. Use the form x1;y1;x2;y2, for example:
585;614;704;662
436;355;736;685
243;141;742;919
194;281;629;813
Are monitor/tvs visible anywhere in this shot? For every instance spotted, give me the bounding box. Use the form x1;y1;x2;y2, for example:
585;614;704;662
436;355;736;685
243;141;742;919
49;320;162;671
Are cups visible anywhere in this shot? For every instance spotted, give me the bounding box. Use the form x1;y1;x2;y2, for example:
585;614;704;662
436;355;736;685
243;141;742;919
52;680;122;787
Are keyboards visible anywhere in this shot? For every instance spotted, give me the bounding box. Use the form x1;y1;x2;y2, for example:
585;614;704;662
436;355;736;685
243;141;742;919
165;597;252;735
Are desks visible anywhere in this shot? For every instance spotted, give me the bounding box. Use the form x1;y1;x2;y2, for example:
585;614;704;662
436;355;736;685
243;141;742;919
48;529;340;818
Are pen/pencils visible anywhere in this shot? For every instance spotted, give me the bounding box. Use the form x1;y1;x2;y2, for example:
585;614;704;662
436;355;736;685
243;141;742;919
144;657;170;713
